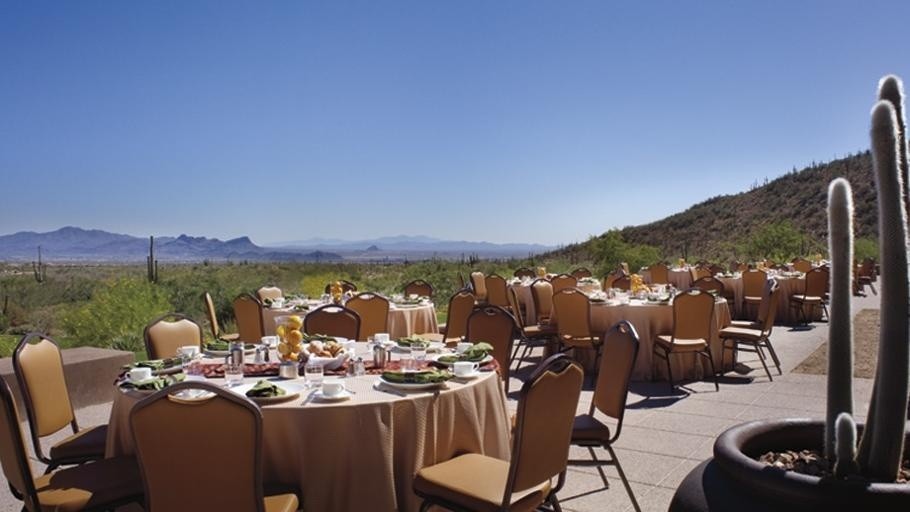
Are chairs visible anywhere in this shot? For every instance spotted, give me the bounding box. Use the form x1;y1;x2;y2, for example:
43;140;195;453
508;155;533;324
412;354;584;512
204;252;733;390
12;332;108;475
511;321;640;512
143;312;202;362
652;289;721;395
129;381;302;512
642;254;878;329
718;280;784;381
1;377;146;512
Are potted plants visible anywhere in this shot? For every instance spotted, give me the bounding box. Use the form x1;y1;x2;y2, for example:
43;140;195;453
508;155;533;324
662;74;910;512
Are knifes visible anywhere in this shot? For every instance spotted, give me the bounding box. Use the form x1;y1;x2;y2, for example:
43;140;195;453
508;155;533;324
300;387;317;406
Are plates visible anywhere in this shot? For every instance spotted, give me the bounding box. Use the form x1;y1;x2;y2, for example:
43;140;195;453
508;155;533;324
378;375;440;391
452;372;479;379
308;352;350;369
314;390;351;400
304;336;347;344
206;344;260;356
395;341;448;353
718;273;804;279
250;389;301;402
440;352;496;367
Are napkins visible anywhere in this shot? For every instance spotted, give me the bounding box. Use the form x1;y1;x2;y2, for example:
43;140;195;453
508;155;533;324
115;357;192;391
245;381;286;399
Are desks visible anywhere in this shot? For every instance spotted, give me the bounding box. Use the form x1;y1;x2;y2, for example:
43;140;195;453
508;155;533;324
110;340;509;512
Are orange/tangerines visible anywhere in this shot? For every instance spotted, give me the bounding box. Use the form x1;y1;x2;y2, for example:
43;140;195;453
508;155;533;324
276;315;303;363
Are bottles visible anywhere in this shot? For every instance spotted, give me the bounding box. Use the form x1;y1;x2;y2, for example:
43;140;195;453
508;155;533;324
346;358;364;377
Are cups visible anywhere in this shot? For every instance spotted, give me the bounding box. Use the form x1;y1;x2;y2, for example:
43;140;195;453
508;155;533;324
453;361;480;374
511;274;621;302
297;288;409;306
304;364;324;388
372;344;392;369
224;365;243;386
262;336;280;346
411;342;427;358
125;345;202;384
323;379;346;396
365;333;391;351
